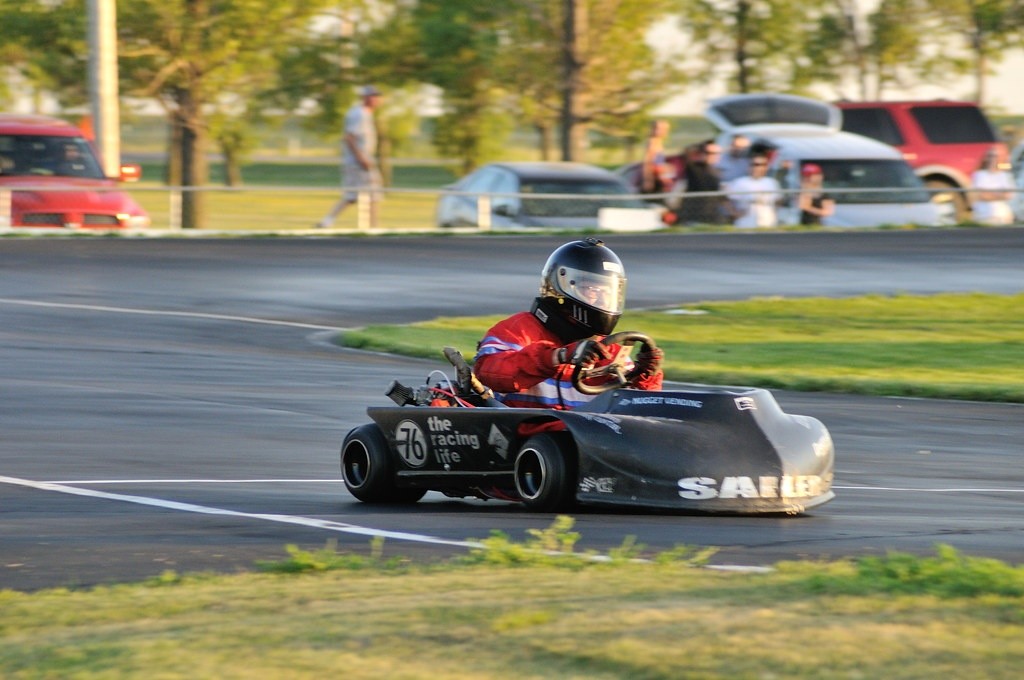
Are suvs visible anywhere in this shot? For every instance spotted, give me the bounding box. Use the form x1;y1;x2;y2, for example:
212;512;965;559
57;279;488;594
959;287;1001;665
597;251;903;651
832;97;1013;223
0;114;154;234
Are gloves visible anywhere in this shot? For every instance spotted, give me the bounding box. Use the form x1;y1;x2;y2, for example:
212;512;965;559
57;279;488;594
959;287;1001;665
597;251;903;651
558;335;611;369
635;344;664;377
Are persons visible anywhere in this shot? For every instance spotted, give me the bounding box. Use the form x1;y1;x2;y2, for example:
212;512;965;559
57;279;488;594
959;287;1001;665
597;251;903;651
967;146;1017;225
54;143;93;175
643;119;835;225
475;236;664;432
317;87;382;231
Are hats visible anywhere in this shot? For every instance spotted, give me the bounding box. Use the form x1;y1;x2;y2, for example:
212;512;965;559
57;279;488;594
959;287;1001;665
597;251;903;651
801;164;822;177
361;87;379;97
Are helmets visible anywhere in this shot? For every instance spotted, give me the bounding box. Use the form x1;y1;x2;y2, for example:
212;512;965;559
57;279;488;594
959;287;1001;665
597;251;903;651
530;237;626;341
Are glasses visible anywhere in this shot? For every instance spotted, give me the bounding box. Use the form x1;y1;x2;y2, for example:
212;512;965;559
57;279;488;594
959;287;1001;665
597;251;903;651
753;163;767;168
704;151;718;157
574;284;615;301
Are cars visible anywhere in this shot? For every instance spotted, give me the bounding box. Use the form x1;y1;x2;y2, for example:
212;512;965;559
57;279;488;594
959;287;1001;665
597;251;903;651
585;156;714;201
432;156;674;231
694;89;957;229
